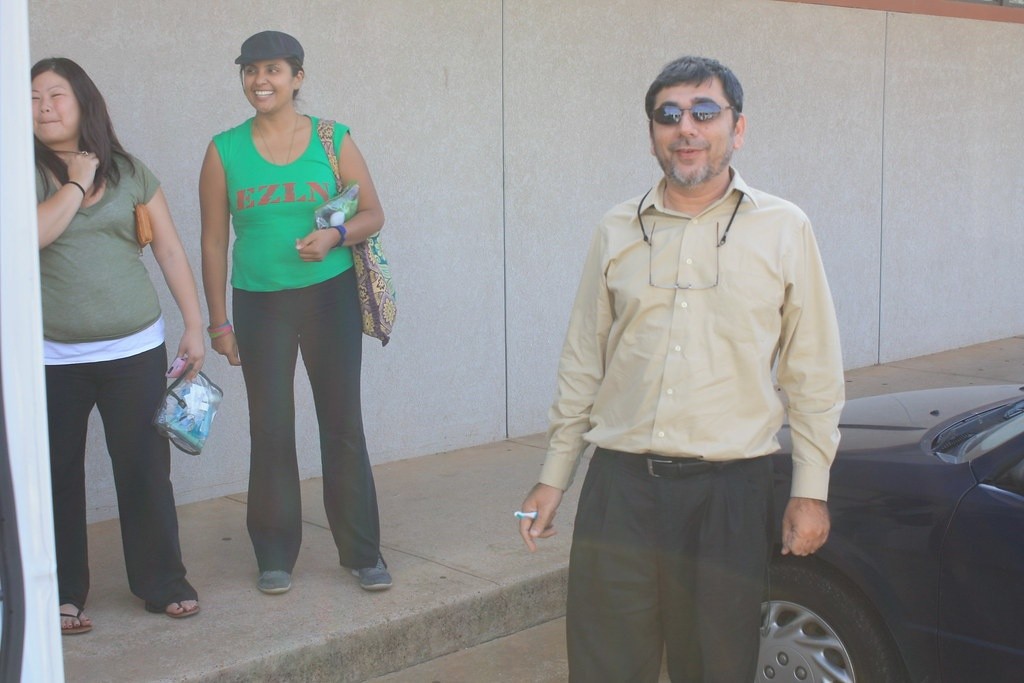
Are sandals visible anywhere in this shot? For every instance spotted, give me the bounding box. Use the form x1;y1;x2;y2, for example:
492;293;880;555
61;607;90;633
164;598;200;618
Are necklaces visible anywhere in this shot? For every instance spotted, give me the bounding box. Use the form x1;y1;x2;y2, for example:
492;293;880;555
254;110;298;166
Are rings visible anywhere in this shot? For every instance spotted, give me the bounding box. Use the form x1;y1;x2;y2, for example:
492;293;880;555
80;151;88;156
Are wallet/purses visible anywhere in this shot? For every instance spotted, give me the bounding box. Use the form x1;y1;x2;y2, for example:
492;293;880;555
136;203;151;245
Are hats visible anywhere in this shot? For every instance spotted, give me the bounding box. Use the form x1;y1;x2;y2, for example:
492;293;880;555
235;31;304;68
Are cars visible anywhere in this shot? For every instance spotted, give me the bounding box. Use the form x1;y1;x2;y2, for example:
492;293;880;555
753;383;1023;683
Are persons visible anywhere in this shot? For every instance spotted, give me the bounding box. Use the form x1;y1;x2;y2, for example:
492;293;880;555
515;55;846;683
31;58;206;635
200;31;392;594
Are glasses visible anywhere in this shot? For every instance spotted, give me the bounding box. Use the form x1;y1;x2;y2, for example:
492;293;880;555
649;219;720;289
652;98;731;125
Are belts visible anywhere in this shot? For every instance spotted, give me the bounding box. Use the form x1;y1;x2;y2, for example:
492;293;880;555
617;451;736;479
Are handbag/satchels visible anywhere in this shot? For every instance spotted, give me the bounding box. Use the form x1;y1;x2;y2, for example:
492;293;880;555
314;180;360;231
353;230;397;346
152;364;223;456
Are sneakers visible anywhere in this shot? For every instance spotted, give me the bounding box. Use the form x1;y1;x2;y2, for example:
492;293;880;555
354;554;393;589
258;568;293;594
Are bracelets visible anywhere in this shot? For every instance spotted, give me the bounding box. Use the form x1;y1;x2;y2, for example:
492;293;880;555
335;225;347;248
206;321;233;340
65;181;85;198
